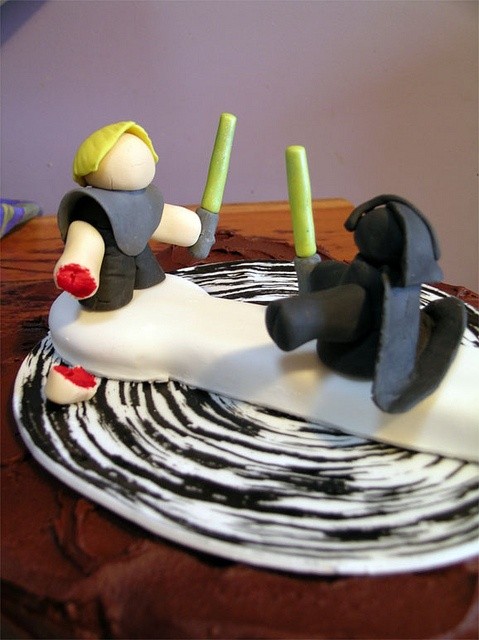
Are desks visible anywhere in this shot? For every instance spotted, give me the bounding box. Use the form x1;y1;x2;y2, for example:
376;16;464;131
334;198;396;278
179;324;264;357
0;198;478;638
